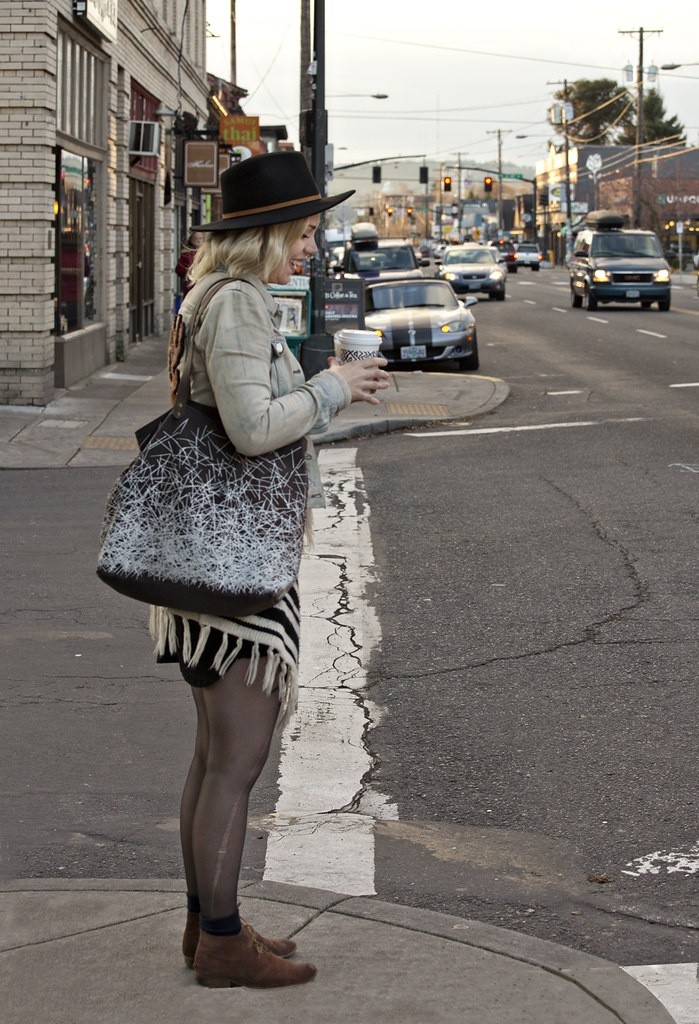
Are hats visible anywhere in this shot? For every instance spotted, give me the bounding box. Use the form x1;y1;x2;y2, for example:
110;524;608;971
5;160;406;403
189;152;356;233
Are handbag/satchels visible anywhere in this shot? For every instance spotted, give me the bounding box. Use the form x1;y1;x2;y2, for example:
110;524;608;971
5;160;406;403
95;278;308;618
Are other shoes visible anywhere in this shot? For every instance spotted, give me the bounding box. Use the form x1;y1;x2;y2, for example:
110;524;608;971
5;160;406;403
174;911;296;958
194;930;316;988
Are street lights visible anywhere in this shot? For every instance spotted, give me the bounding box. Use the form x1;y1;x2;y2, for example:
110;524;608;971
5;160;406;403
517;132;575;269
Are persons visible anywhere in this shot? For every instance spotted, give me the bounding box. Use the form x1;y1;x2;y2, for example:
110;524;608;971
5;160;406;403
176;231;205;299
149;152;389;990
61;231;91;327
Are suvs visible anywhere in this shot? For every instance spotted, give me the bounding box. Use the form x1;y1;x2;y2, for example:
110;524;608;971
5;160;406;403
330;222;430;310
513;244;543;271
492;240;520;274
570;209;673;312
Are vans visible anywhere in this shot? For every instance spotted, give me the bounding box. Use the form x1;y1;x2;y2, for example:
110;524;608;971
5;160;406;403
323;229;353;273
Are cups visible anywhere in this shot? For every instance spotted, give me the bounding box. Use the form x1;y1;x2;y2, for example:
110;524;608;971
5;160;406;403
337;328;382;398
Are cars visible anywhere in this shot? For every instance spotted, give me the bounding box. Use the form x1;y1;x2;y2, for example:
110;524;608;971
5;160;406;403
356;277;480;376
430;245;507;303
414;243;448;264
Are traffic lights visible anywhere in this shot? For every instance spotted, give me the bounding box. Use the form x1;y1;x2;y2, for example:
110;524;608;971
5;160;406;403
369;207;374;216
485;177;493;193
444;177;452;193
407;208;413;217
388;208;393;216
